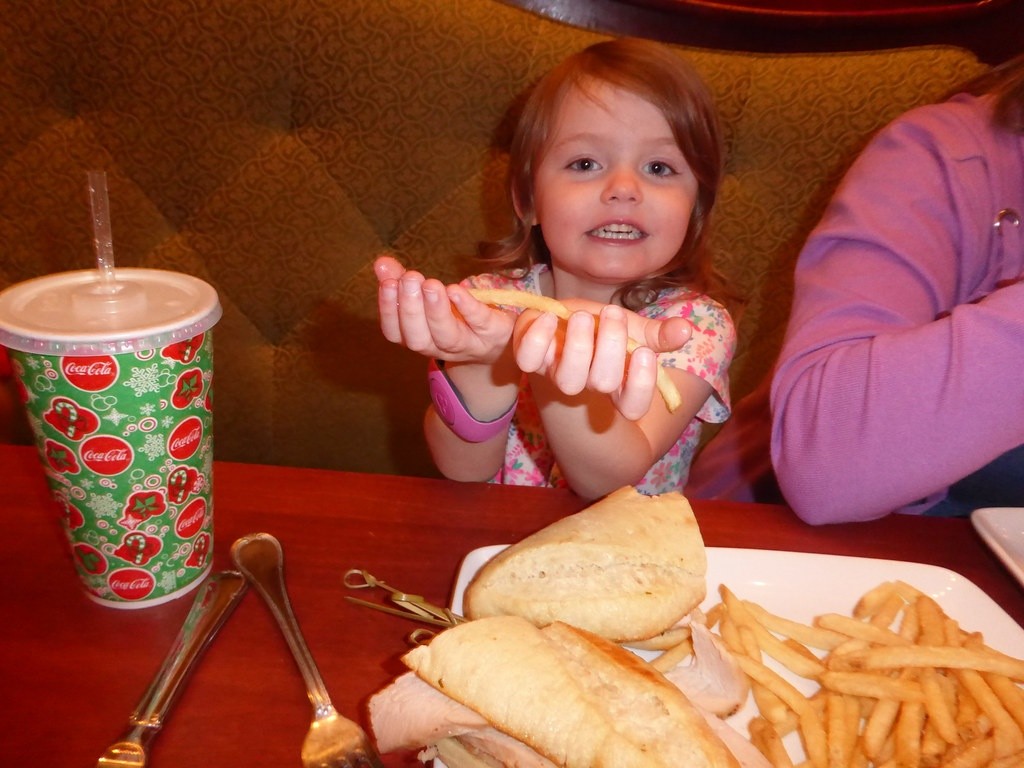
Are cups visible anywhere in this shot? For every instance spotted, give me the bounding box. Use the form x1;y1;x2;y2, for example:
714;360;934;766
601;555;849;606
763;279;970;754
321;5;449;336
0;267;224;608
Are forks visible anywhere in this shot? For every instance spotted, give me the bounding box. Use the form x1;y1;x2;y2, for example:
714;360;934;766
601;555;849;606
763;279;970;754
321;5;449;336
230;532;385;768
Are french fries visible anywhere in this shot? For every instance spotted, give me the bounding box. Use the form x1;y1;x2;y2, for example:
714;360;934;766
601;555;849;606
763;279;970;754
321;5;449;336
627;337;683;413
621;579;1024;768
467;286;573;319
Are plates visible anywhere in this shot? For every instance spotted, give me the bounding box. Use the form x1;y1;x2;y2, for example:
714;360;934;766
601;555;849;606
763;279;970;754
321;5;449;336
432;545;1024;768
971;507;1023;584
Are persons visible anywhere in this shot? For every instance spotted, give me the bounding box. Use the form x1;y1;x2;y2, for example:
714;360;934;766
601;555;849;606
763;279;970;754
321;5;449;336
769;55;1024;526
373;38;737;501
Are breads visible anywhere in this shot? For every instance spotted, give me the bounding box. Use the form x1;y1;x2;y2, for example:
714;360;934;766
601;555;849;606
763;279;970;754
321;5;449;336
462;486;707;637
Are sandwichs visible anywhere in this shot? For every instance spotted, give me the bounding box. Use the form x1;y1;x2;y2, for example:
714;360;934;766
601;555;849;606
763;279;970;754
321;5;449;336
367;616;741;768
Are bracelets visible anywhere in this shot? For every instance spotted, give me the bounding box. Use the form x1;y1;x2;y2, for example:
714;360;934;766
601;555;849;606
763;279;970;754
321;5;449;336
426;358;518;443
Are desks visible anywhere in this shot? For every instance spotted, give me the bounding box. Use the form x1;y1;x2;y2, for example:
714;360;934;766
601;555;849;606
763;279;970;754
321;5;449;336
0;445;1023;768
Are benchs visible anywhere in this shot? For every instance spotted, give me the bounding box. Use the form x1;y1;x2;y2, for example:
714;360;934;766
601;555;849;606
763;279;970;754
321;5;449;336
0;2;1023;478
